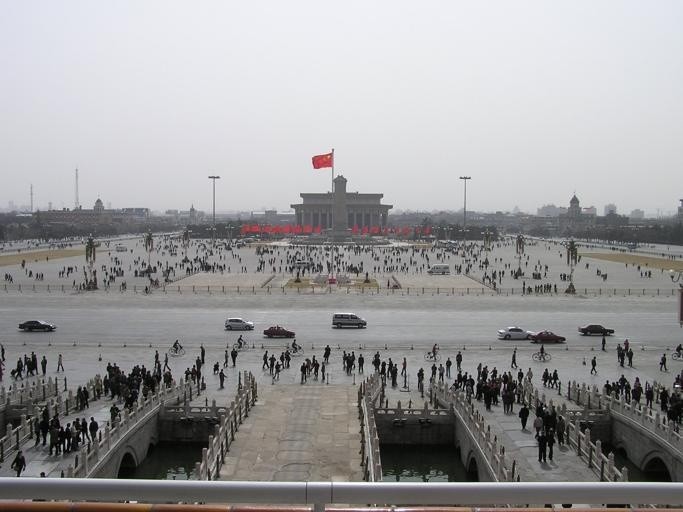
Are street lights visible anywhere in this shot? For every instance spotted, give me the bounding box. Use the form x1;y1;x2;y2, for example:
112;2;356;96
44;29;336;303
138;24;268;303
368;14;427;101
207;175;219;246
459;176;471;248
668;267;682;324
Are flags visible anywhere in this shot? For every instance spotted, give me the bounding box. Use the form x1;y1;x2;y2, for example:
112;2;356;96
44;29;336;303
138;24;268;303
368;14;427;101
311;153;333;169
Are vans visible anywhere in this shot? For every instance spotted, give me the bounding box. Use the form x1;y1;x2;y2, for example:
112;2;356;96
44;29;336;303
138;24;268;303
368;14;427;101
331;311;367;330
628;243;637;250
560;240;572;246
426;264;450;275
295;261;311;269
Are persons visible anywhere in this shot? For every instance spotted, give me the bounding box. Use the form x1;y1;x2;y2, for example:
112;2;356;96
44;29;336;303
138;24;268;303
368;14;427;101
604;374;681;432
372;351;407;387
526;367;559;388
417;344;462;392
2;226;249;293
617;339;633;368
676;344;681;357
1;334;247;481
511;351;519;368
478;227;681;293
342;350;364;376
591;356;597;374
539;344;546;360
301;355;325;385
256;226;477;289
601;336;606;350
323;345;332;364
519;403;566;462
450;363;524;414
262;339;299;379
660;354;668;371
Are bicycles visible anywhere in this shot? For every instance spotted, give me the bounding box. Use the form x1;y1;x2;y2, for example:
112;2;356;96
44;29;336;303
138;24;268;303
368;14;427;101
166;344;185;357
423;347;442;361
288;343;304;354
530;350;553;362
232;341;249;351
669;350;682;362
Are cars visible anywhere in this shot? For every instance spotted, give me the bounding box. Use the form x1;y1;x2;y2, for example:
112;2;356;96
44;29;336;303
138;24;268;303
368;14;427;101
224;318;254;330
18;318;56;332
494;322;616;345
262;325;295;339
